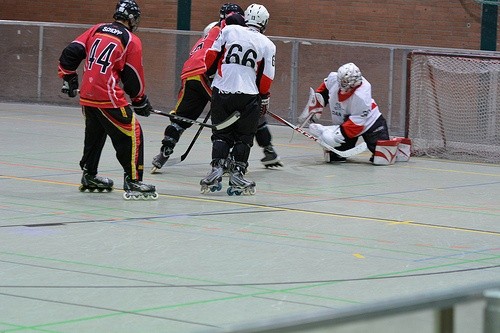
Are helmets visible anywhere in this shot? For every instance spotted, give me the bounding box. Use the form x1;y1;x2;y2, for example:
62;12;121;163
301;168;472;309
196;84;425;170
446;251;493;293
337;63;362;92
113;0;141;32
220;2;232;18
244;4;269;33
225;4;244;15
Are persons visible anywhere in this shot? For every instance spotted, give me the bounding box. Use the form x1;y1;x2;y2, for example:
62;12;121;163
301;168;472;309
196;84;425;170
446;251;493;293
58;0;158;200
299;63;411;165
200;4;276;195
151;3;283;175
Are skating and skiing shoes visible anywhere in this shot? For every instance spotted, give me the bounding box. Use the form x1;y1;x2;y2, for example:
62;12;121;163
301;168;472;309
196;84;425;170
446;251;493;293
200;159;225;194
223;152;248;177
79;163;113;193
123;169;158;200
149;145;173;174
227;160;257;196
260;144;284;168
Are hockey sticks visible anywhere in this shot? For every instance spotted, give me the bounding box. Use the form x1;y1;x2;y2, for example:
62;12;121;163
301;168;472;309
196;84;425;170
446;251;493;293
76;89;240;131
164;111;210;166
296;113;314;129
265;110;367;157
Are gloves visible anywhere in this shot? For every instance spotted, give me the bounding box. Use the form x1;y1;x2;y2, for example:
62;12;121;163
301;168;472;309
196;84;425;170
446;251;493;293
258;93;271;118
62;73;78;97
132;95;152;116
